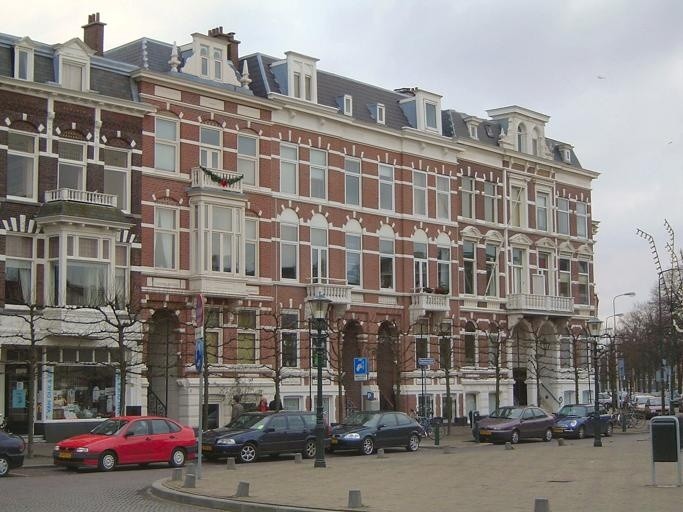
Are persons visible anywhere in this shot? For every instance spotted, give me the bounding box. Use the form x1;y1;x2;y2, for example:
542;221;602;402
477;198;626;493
257;394;282;411
232;395;244;422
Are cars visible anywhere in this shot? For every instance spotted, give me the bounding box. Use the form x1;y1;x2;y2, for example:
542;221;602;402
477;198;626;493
0;429;26;476
473;391;683;444
53;415;199;473
331;410;426;455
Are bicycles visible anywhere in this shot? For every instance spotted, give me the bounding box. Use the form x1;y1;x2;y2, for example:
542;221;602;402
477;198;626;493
416;417;446;440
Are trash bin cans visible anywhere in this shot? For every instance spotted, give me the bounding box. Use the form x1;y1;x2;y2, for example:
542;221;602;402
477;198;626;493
469;411;479;428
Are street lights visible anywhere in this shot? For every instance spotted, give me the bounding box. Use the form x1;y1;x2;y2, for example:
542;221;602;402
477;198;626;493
605;291;635;335
307;292;332;468
585;317;603;447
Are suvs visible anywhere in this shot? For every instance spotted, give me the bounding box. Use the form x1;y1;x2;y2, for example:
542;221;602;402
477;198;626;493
201;410;331;463
